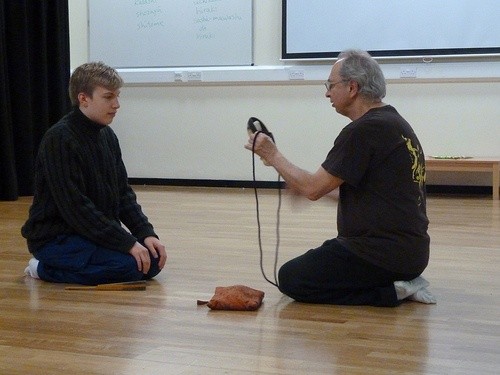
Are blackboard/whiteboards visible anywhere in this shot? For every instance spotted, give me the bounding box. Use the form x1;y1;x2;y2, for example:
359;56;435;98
85;0;256;67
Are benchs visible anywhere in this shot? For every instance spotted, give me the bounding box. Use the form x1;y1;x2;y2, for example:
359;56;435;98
424;157;500;200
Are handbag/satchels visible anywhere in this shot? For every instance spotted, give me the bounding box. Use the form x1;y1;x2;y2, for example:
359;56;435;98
196;285;264;311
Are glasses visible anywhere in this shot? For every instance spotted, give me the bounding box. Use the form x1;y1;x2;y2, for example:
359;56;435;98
324;80;347;91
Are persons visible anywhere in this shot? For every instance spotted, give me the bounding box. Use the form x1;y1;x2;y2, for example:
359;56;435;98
245;49;438;306
21;61;168;285
